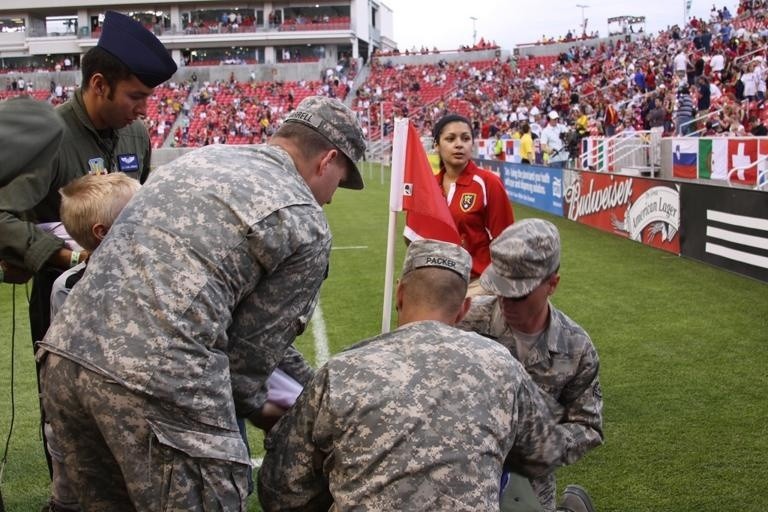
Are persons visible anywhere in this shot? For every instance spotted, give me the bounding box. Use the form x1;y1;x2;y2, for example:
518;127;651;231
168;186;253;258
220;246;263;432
34;95;365;512
456;217;605;512
406;115;515;283
258;238;570;512
49;171;142;327
0;10;178;486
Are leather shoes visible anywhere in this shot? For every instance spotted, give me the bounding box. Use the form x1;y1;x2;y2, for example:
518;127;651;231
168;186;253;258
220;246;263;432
557;484;595;512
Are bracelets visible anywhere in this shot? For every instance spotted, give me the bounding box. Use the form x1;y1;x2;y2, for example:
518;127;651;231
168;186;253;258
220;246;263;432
70;251;80;267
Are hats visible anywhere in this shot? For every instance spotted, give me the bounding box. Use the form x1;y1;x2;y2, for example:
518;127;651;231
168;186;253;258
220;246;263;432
283;95;368;189
402;239;473;285
479;217;561;299
752;56;762;62
97;9;177;87
547;110;559;121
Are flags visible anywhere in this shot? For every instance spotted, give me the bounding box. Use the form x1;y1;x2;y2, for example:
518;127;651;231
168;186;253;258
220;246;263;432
389;115;461;246
